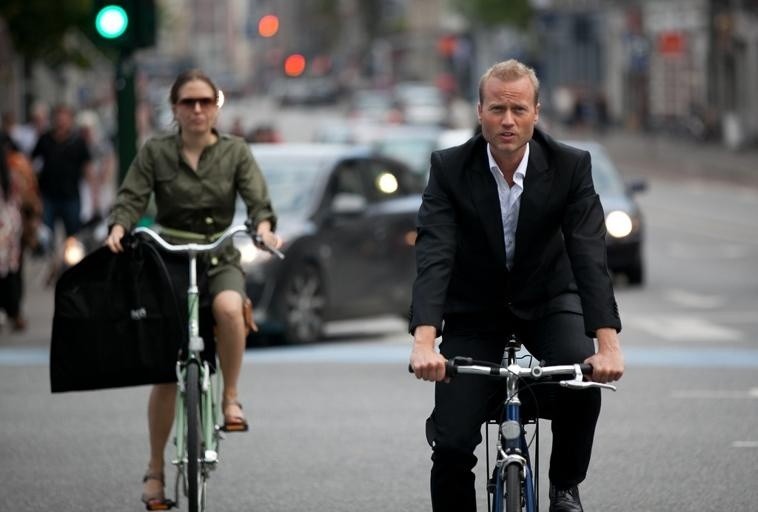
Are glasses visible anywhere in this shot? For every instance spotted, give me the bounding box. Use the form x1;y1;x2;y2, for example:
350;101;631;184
175;97;214;107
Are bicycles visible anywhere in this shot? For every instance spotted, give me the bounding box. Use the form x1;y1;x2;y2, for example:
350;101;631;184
122;217;287;511
401;331;619;511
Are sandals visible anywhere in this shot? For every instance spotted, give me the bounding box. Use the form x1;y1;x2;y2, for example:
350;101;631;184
140;471;164;506
222;400;246;425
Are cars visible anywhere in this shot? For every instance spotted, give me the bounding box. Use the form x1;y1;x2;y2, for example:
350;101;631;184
59;138;429;346
265;76;475;169
434;130;657;288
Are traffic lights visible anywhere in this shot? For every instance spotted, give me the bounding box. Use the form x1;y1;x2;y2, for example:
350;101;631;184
85;4;157;61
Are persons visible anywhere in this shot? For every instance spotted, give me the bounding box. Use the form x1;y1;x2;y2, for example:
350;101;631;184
0;83;103;332
407;59;618;512
103;69;279;507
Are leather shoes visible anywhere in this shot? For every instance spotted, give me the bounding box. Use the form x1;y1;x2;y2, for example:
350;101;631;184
548;482;582;512
